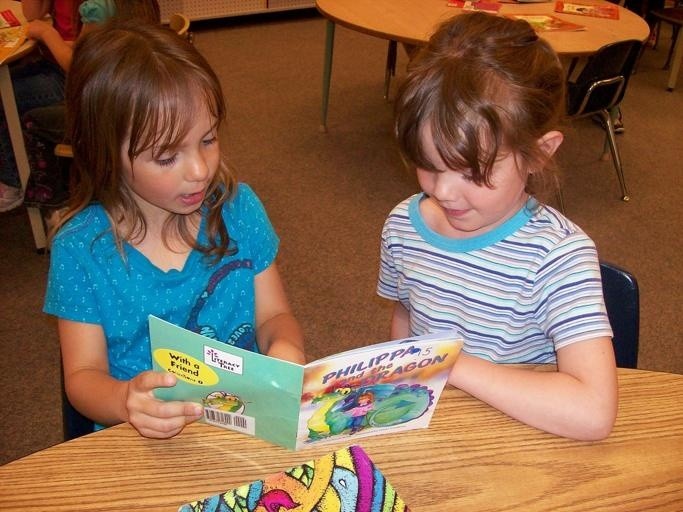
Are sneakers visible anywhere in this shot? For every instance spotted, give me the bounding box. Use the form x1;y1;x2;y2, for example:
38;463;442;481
592;106;625;133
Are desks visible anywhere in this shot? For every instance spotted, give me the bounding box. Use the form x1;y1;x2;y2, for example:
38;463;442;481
310;1;649;140
0;356;683;512
0;0;64;256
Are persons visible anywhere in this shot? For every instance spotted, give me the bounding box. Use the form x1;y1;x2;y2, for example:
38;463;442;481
0;1;84;214
20;0;160;250
41;13;309;439
590;102;626;134
376;11;619;443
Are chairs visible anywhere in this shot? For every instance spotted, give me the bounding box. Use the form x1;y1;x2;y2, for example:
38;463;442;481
550;40;644;219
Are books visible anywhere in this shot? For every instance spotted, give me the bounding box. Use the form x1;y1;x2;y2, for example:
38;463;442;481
553;0;621;19
459;0;501;16
502;12;587;35
146;313;463;454
